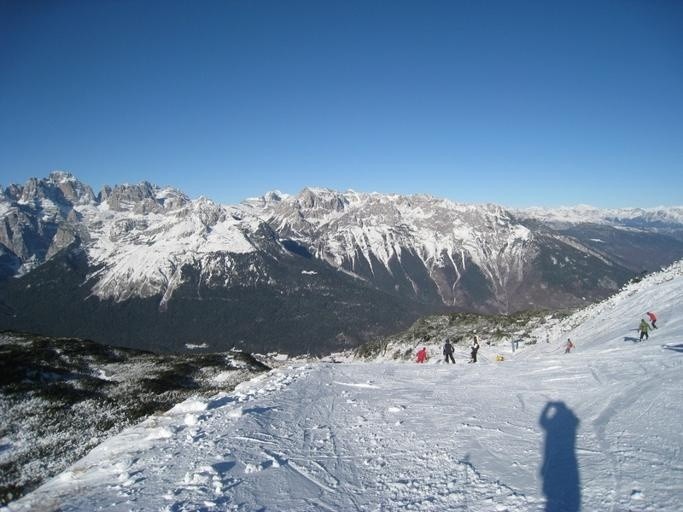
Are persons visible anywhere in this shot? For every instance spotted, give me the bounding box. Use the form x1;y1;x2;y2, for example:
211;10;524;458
647;312;657;329
638;319;652;342
468;336;479;362
415;347;427;363
563;338;573;353
443;339;456;364
